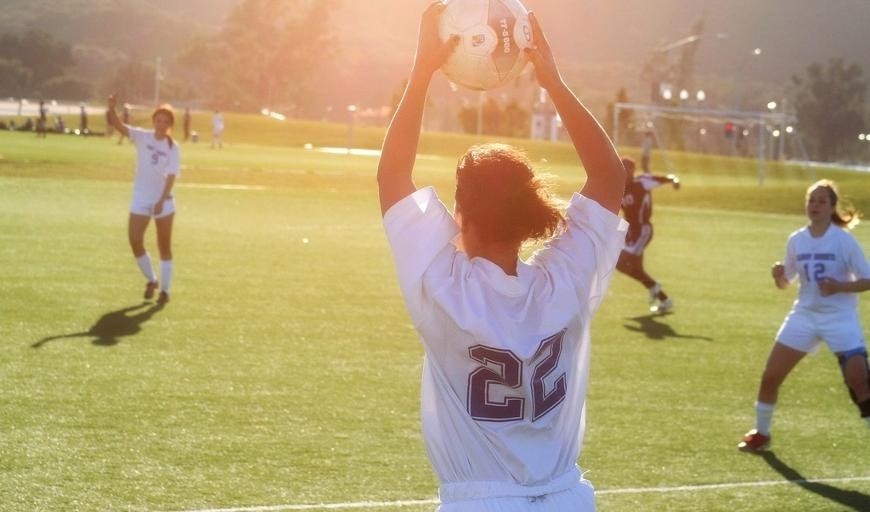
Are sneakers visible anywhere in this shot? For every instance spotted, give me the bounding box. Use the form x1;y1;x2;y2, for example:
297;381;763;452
649;282;673;313
738;430;771;451
145;280;169;304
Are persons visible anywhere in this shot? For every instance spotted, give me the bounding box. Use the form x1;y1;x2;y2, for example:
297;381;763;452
103;92;183;307
617;157;681;314
738;179;869;454
639;130;654;174
0;99;226;151
374;1;625;509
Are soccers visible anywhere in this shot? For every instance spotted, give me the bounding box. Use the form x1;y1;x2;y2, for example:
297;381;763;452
437;0;535;91
